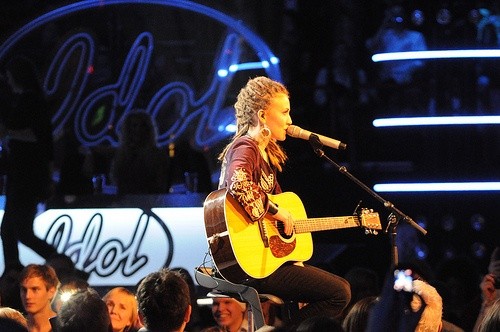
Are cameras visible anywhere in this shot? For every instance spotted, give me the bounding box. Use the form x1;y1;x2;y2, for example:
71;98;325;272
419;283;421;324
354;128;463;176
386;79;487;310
493;276;500;289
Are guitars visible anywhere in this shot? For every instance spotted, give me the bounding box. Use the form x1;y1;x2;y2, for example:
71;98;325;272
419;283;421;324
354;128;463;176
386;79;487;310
203;188;382;286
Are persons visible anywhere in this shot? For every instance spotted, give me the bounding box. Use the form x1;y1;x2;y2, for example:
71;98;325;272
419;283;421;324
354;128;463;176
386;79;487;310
0;256;250;332
219;76;352;332
258;213;500;332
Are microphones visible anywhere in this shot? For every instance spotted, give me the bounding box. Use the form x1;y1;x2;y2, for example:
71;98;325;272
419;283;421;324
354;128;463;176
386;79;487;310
286;125;347;150
